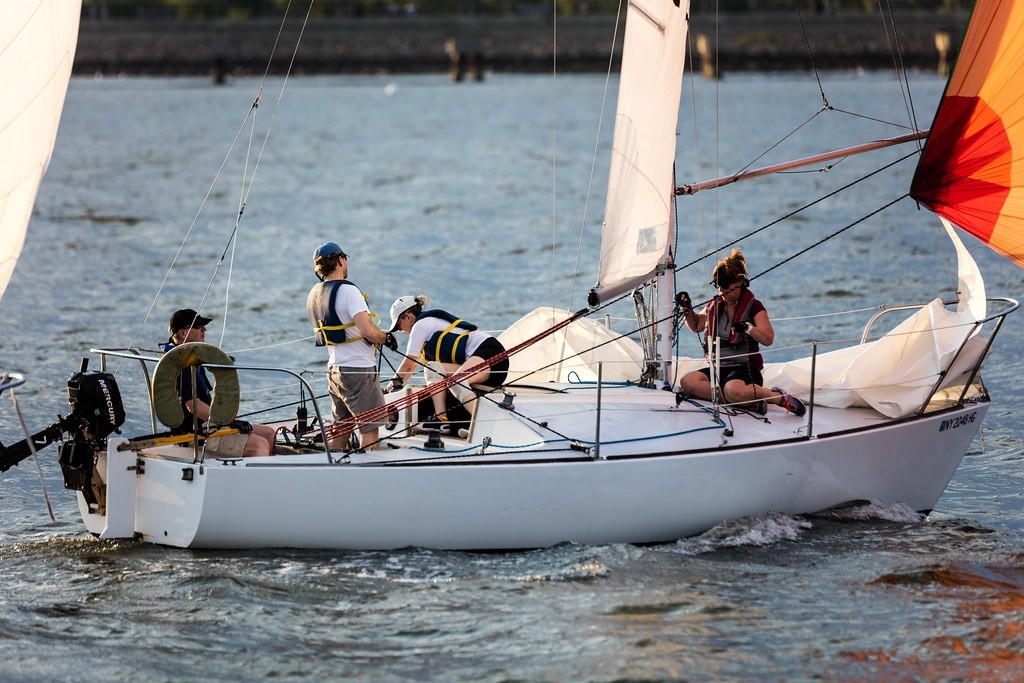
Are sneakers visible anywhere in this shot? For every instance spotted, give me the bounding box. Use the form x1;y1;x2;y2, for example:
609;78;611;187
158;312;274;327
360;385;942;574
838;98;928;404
458;428;469;438
749;400;767;414
771;385;807;417
410;414;451;434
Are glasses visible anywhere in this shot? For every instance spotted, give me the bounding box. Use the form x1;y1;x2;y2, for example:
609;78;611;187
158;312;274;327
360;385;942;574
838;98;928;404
183;326;204;330
709;280;729;289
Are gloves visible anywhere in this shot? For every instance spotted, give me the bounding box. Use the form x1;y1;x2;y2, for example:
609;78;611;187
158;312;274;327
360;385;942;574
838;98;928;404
230;420;253;435
385;332;398;351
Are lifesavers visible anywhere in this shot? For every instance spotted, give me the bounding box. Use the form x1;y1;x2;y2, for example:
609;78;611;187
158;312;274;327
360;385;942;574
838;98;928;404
152;342;240;428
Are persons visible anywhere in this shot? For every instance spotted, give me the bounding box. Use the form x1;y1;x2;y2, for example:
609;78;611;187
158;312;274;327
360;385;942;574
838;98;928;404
384;295;510;434
673;249;807;417
165;308;277;458
307;243;398;450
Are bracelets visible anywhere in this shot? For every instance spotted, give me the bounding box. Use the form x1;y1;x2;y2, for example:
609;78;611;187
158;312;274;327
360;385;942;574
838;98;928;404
745;321;753;335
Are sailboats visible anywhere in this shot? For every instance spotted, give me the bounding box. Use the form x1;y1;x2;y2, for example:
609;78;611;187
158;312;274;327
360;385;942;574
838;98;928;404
1;1;995;552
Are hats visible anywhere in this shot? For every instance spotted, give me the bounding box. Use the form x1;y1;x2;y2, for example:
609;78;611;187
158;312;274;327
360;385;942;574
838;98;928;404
170;309;213;333
313;243;350;265
710;272;746;286
390;295;418;332
